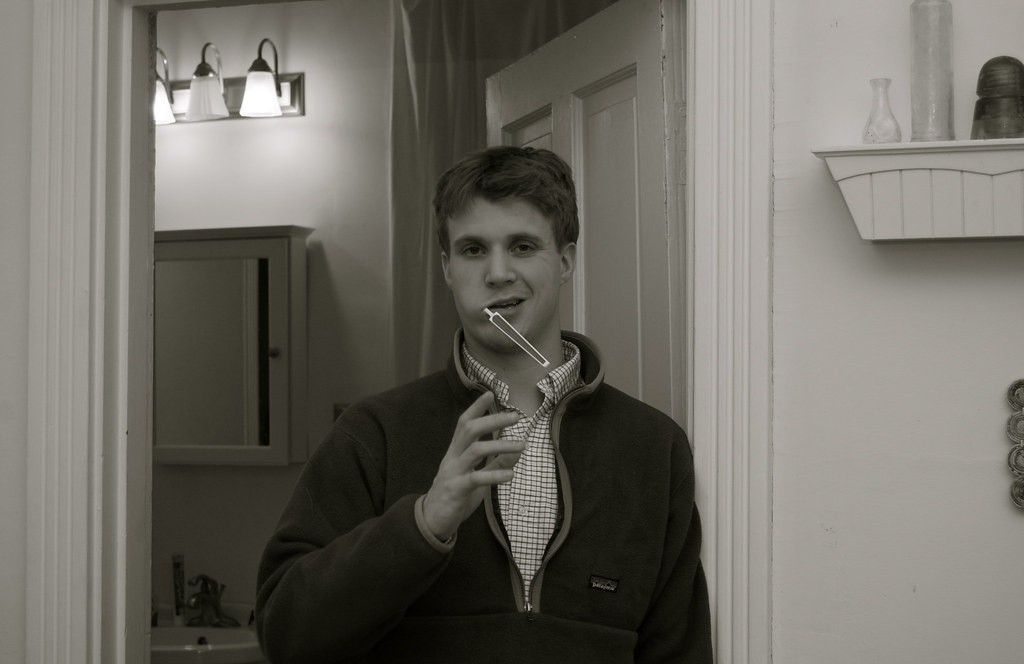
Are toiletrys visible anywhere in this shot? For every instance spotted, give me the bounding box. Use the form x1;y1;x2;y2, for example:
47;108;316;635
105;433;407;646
172;553;186;626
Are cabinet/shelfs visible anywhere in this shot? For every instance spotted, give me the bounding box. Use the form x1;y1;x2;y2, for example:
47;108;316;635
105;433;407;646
153;224;310;465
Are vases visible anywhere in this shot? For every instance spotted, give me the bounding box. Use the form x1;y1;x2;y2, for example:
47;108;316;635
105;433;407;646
861;78;903;145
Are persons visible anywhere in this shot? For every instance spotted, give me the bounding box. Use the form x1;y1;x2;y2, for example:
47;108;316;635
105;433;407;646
254;144;715;664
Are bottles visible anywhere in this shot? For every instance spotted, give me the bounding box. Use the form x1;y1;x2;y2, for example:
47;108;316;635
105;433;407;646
909;0;960;140
862;77;901;145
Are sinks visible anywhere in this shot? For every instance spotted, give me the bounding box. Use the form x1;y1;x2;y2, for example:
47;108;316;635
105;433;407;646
151;625;266;664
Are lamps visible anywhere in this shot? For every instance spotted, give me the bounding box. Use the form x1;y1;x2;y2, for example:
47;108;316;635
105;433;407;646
182;41;230;122
152;46;176;126
240;38;285;119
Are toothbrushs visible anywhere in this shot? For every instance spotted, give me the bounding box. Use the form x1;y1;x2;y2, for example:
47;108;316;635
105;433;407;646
483;308;551;368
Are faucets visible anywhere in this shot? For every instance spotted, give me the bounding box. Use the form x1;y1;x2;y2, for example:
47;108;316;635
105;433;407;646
186;574;242;628
